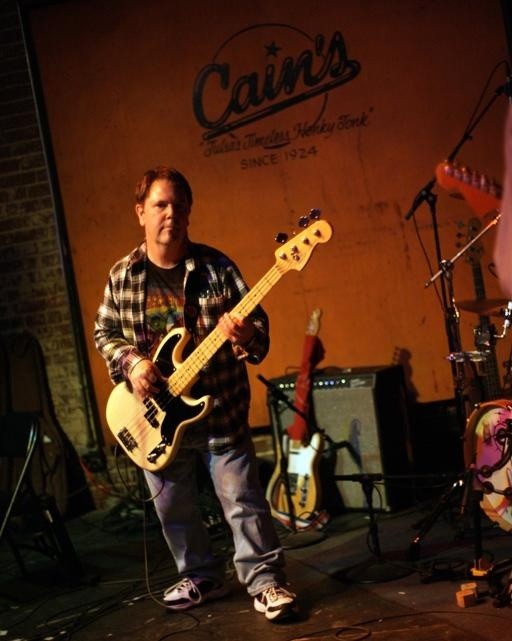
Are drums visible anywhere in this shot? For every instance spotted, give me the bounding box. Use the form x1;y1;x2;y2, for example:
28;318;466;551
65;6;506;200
459;399;512;535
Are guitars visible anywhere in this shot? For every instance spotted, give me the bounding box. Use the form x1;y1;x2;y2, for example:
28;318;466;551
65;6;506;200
263;308;338;533
452;216;510;402
104;208;334;474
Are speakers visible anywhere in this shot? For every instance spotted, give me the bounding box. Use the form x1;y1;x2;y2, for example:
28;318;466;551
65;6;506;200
266;364;408;512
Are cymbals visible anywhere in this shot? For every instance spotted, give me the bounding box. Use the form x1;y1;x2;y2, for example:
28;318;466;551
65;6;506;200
455;298;510;316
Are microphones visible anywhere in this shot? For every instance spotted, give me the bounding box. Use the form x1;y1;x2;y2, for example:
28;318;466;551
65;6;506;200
505;63;512;104
318;441;349;455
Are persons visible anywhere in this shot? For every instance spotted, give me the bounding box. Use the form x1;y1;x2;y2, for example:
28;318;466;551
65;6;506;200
90;166;301;625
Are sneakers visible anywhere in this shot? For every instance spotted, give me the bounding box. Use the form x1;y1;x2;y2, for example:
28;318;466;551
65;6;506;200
254;585;298;620
164;578;228;610
